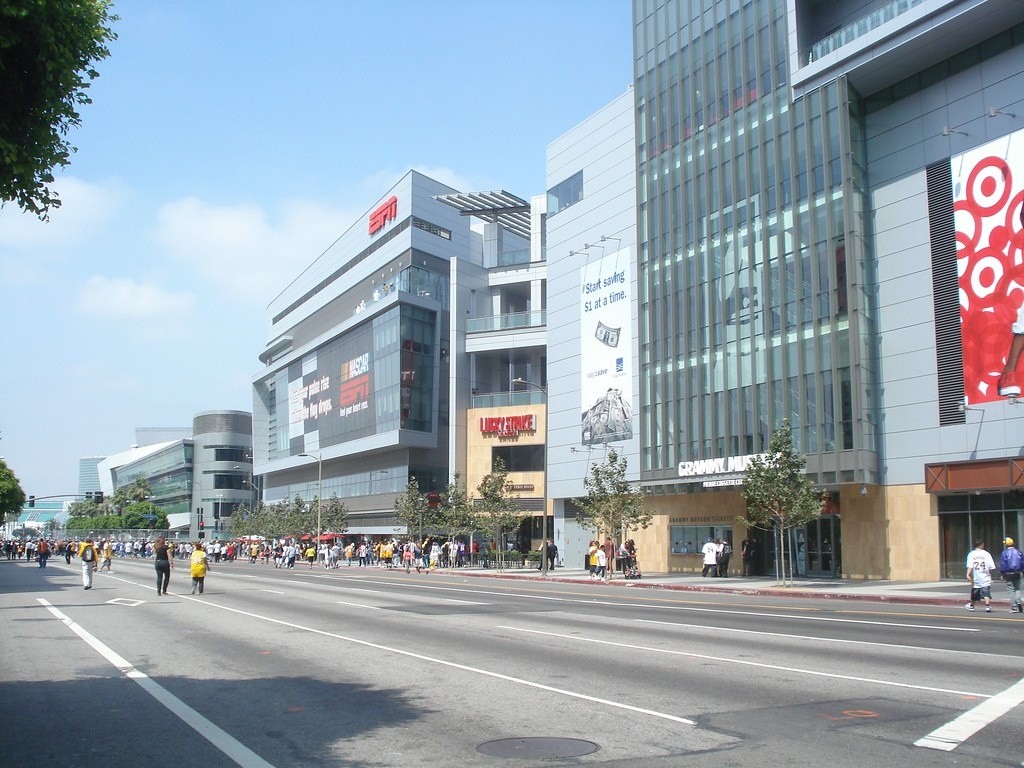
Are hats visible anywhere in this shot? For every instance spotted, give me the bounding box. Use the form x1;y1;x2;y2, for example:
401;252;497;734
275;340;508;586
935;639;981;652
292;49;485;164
1003;537;1013;547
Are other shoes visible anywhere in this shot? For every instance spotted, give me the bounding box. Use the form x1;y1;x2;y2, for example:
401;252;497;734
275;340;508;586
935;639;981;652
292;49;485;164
1018;604;1023;612
1008;608;1018;613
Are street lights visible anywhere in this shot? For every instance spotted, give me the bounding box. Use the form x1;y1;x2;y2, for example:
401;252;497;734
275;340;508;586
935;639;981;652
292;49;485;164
298;452;322;564
418;498;424;553
242;479;260;504
510;378;549;577
145;496;155;516
185;480;202;542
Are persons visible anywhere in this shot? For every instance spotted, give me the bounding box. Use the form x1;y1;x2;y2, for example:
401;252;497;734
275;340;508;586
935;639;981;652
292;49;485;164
474;539;496;568
165;541;203;560
38;539;48;568
700;537;732;578
588;536;638;581
79;537;98;590
324;542;341;570
740;537;762;575
0;538;79;564
251;542;296;569
190;544;211;595
95;538;114;573
537;540;557;571
999;537;1024;614
359;540;466;569
109;540;157;559
965;539;996;612
298;543;316;569
154;536;175;596
344;542;353;566
206;540;242;564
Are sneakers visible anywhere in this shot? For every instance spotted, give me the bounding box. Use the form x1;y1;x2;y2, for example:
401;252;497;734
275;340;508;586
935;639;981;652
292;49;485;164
985;606;992;612
965;603;975;612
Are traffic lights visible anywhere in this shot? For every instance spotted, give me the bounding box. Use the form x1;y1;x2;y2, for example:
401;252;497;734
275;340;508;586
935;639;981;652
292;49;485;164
200;522;204;531
